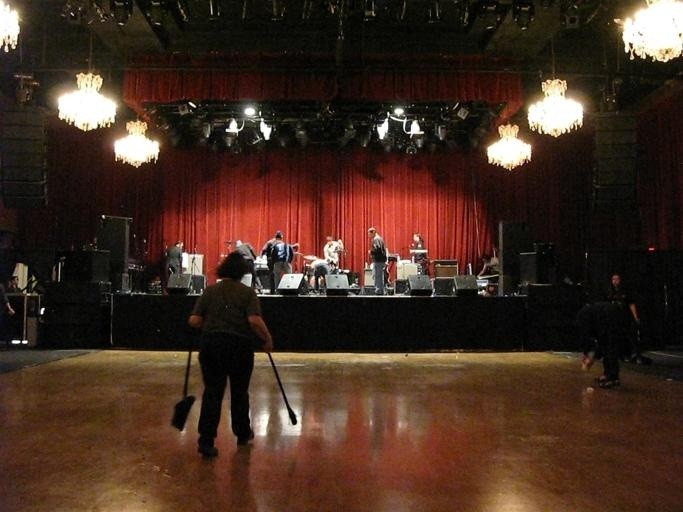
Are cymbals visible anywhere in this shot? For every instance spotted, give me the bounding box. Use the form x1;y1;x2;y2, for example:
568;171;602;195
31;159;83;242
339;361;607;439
333;249;345;253
303;255;318;260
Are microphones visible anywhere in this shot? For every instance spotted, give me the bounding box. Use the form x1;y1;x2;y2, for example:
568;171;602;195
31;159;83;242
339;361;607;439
225;241;236;245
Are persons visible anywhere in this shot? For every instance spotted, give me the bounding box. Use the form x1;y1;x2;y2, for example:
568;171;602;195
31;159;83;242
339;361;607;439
476;253;500;278
164;226;432;294
604;272;652;365
0;276;25;345
576;299;631;387
310;257;330;291
188;250;273;457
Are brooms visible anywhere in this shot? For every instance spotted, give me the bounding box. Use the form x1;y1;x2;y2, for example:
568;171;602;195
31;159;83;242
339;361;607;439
267;349;297;425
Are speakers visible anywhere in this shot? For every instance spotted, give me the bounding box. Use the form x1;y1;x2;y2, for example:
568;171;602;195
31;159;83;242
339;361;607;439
166;272;192;294
75;247;110;292
408;274;432;293
98;213;133;289
6;292;41;342
454;272;479;293
276;272;305;294
324;274;350;295
497;219;528;296
519;250;548;282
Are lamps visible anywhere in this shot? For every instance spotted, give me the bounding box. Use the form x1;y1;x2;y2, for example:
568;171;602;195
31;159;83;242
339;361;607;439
486;37;584;174
619;0;682;64
54;30;161;170
67;0;536;33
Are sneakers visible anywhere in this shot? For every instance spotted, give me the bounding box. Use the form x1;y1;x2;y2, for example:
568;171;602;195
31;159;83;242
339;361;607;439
595;376;620;388
198;430;218;456
238;430;254;445
